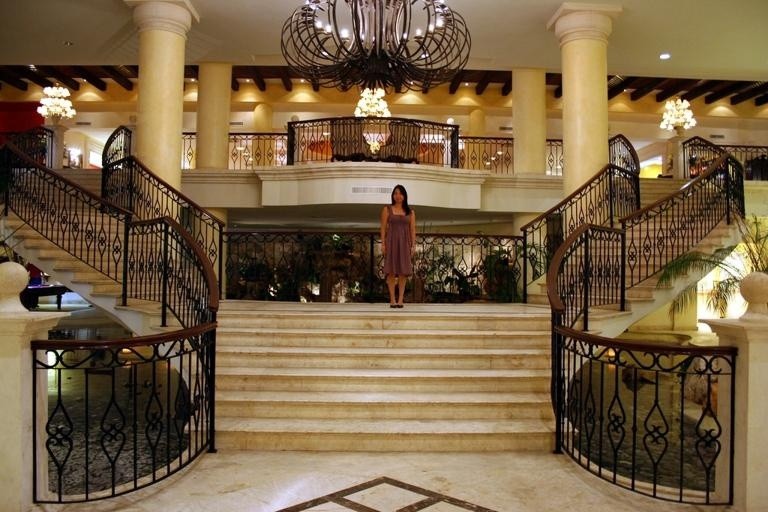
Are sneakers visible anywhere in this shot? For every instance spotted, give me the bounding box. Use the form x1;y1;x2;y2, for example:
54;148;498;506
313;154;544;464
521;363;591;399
390;304;403;308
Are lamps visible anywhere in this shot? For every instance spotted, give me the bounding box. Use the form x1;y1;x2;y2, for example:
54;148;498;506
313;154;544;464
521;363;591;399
280;0;472;96
659;94;697;134
37;78;77;124
354;85;391;153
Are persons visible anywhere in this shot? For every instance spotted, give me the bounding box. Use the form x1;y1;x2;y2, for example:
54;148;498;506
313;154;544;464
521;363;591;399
380;184;417;308
26;262;45;286
